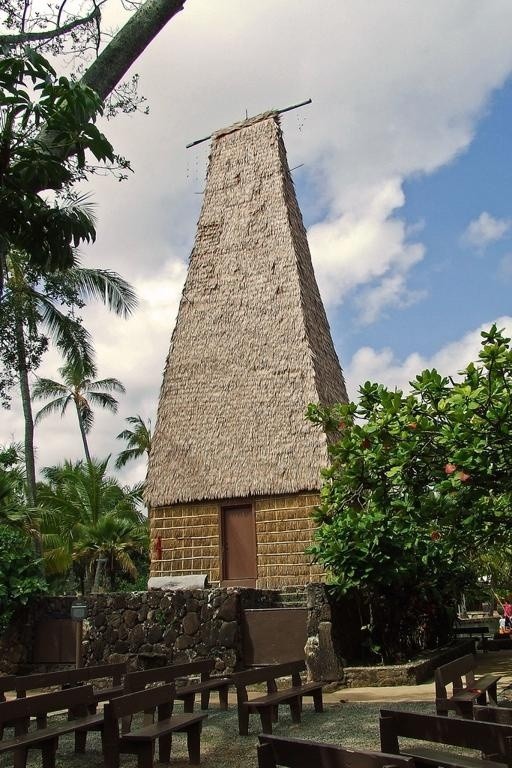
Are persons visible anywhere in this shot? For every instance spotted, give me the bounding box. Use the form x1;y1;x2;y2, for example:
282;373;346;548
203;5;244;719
499;615;506;634
501;601;512;631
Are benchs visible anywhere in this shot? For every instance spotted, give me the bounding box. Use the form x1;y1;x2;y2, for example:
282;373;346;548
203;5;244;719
1;657;322;768
257;654;511;766
